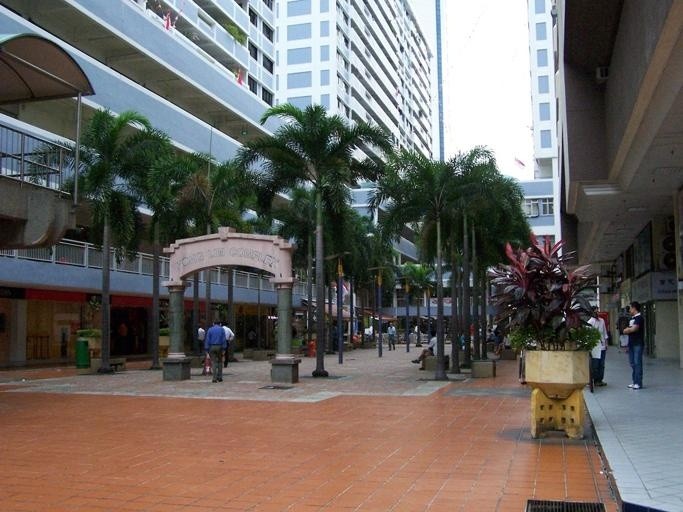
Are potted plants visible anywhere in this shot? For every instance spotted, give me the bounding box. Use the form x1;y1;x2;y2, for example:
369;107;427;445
486;236;602;397
77;295;102;356
158;328;167;355
291;337;302;354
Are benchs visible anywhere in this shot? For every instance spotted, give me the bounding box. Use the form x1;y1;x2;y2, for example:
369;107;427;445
110;362;124;370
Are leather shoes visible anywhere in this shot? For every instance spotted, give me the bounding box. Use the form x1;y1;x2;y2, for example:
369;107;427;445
594;381;607;386
218;379;222;382
212;380;217;383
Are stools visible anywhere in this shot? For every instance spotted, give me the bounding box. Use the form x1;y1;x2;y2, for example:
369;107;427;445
424;355;450;372
486;342;494;351
500;349;515;359
457;349;470;365
471;361;495;378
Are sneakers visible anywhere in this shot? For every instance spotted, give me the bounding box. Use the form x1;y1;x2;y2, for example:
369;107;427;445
633;384;642;389
628;384;633;388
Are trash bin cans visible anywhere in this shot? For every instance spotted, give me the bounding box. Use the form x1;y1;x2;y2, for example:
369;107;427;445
75;338;90;368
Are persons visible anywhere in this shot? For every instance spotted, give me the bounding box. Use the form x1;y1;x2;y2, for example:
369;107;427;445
203;318;228;383
330;325;338;351
195;323;205;355
623;301;645;390
457;321;535;386
411;329;437;369
586;303;608;386
386;322;396;351
219;321;236;368
